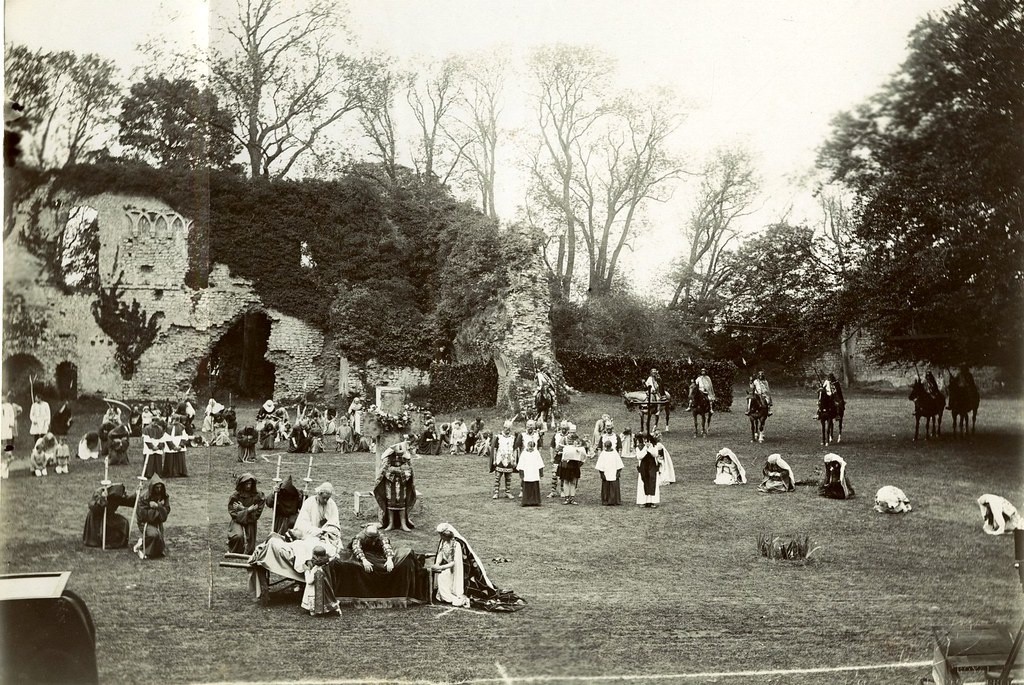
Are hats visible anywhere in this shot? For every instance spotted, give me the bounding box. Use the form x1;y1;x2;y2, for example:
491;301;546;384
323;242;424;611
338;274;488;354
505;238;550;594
263;399;276;414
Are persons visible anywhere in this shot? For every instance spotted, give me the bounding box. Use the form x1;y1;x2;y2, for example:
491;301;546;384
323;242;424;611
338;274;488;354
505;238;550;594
382;392;398;410
430;519;526;616
347;521;397;582
133;470;173;562
0;352;986;515
302;546;345;618
382;443;414;533
224;466;346;583
976;490;1024;574
82;480;143;553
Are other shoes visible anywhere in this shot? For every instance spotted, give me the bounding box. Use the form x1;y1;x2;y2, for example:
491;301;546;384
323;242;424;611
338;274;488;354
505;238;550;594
563;499;570;505
504;492;514;499
547;492;557;498
686;407;691;412
650;504;657;509
710;409;715;414
571;498;579;505
492;493;500;499
639;503;646;508
655;410;661;415
519;492;524;498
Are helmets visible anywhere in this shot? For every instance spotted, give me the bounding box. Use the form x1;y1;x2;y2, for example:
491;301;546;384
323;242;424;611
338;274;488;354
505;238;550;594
365;525;381;538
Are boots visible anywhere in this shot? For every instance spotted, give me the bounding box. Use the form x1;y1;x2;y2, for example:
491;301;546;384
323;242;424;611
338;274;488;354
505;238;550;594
400;510;413;533
385;508;395;532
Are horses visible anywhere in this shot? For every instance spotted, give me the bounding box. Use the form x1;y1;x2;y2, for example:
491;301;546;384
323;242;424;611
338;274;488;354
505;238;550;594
686;383;713;439
816;385;845;447
909;377;946;443
947;372;981;438
743;384;768;444
622;390;672;434
534;381;556;433
